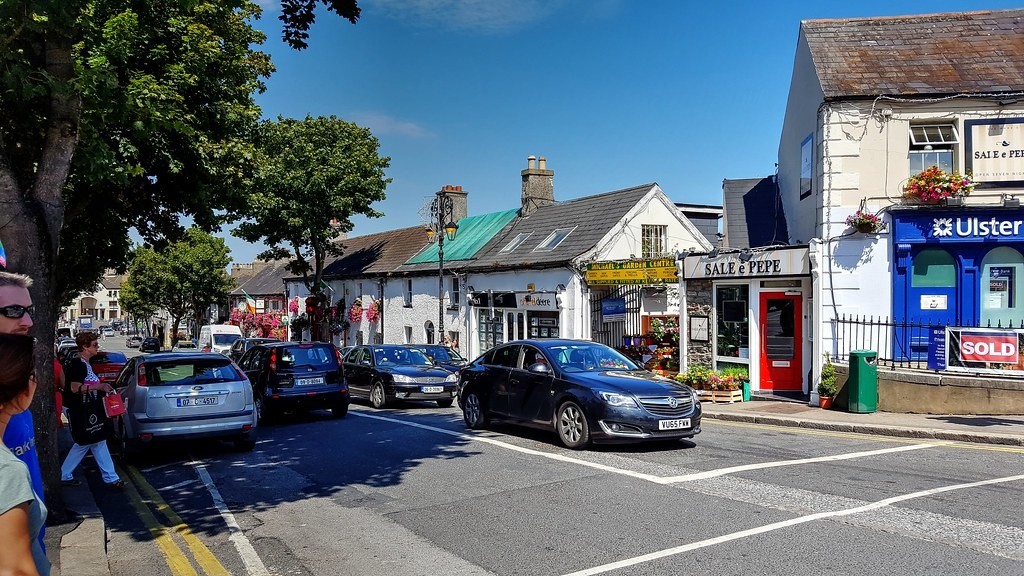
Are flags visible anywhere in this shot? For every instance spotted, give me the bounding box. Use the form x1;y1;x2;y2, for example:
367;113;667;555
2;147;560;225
245;293;255;314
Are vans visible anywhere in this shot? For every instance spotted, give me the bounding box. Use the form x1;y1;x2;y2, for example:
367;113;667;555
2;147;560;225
199;325;242;357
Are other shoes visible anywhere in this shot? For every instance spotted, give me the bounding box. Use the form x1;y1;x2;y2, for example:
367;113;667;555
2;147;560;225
62;479;82;486
105;479;129;488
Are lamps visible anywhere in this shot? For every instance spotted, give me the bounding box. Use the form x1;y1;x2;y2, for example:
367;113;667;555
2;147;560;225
468;291;482;306
738;246;754;264
1004;194;1019;208
1000;95;1018;107
525;289;538;303
495;291;504;303
679;247;698;259
944;196;967;206
709;247;719;258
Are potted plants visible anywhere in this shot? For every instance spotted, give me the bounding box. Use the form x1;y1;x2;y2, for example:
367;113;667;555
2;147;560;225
817;351;837;409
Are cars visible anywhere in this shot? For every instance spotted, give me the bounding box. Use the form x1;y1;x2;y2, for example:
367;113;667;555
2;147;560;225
111;352;258;461
235;339;350;428
404;343;468;377
56;321;80;368
96;320;131;337
457;338;703;452
342;343;459;410
336;345;357;357
89;352;130;384
172;340;198;353
126;336;143;348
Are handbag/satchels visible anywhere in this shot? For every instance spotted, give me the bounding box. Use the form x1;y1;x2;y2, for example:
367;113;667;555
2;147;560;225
67;384;126;445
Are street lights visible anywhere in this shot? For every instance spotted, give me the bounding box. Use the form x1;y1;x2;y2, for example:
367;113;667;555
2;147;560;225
424;191;459;343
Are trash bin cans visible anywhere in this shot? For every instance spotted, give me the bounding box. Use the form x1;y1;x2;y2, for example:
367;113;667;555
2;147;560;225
848;351;879;413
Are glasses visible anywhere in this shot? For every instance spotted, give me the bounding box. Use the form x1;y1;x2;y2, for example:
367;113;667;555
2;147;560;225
87;342;98;348
0;303;36;319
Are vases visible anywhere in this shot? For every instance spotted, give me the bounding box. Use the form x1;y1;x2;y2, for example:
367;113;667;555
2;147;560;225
857;218;874;234
623;337;739;390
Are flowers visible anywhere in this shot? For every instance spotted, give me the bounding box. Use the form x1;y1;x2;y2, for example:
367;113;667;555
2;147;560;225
230;305;284;338
900;163;979;205
619;319;746;384
350;298;364;321
290;296;299;315
846;209;888;228
367;299;383;323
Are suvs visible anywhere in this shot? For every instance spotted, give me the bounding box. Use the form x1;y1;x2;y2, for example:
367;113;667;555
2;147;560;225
138;337;160;352
228;337;281;363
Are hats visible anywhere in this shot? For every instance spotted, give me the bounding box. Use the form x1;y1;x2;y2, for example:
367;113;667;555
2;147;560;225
535;352;545;360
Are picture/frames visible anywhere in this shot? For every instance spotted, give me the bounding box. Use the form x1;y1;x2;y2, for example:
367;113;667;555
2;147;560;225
690;315;710;344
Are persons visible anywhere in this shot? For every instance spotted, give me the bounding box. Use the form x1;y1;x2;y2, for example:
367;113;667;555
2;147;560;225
443;336;460;354
0;273;47;555
247;341;256;349
177;335;182;340
535;353;550;369
60;332;128;493
99;326;106;341
203;342;211;349
53;357;65;425
190;335;197;344
0;332;51;576
